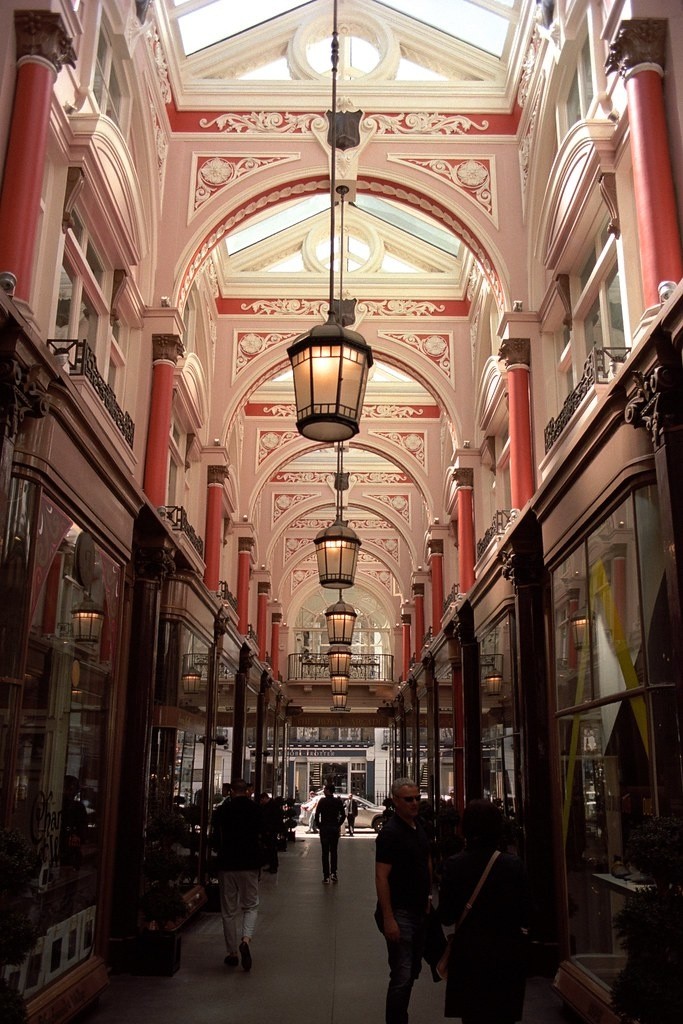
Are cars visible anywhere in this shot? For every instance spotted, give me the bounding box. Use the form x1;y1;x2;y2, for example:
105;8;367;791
299;794;385;833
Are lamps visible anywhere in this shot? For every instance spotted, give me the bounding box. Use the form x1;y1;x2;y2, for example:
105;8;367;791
276;1;379;712
71;585;104;645
480;661;503;695
182;633;202;695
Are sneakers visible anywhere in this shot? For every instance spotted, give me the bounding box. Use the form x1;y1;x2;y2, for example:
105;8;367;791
331;873;338;881
322;877;330;884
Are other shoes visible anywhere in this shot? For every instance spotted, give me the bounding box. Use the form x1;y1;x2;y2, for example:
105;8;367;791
263;866;277;873
313;829;318;833
306;830;311;834
239;942;252;971
225;955;238;966
350;833;353;836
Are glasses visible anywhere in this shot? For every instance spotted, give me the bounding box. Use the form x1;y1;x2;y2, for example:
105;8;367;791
395;795;420;802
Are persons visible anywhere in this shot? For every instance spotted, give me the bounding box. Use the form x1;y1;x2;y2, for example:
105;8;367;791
315;784;346;883
59;776;88;851
437;800;538;1024
211;778;263;970
374;778;435;1024
381;799;393;826
258;792;283;873
301;791;319;833
337;793;358;836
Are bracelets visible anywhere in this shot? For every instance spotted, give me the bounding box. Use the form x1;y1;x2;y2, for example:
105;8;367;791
428;895;434;900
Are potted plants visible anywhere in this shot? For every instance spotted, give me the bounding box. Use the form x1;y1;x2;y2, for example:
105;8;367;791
133;811;190;977
200;810;221;913
283;797;298;842
274;795;288;852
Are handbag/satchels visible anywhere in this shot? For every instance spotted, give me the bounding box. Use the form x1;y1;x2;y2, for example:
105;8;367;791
422;903;448;982
435;934;456;981
